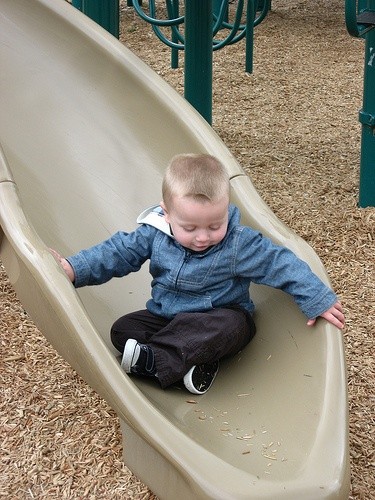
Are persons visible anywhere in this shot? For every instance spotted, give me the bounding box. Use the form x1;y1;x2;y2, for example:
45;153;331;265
44;151;349;397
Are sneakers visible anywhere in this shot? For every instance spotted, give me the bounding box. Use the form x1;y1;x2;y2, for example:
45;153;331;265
120;339;160;381
175;358;220;395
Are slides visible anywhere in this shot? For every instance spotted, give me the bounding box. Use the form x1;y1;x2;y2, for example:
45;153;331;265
2;0;346;500
